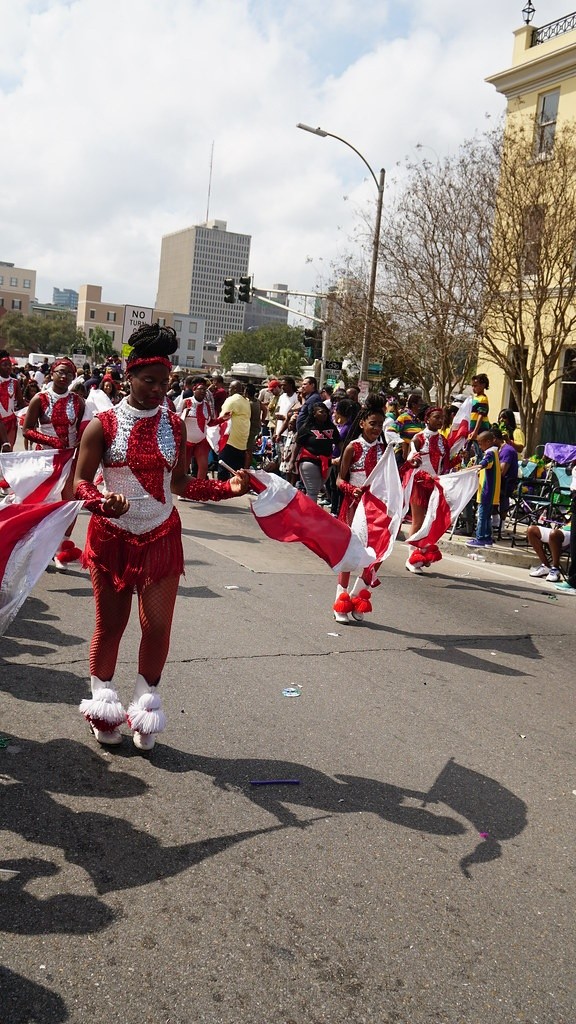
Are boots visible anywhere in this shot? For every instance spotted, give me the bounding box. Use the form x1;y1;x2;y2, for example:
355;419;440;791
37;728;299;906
80;674;127;745
126;673;166;751
404;541;442;574
349;578;372;622
334;584;349;623
54;536;82;571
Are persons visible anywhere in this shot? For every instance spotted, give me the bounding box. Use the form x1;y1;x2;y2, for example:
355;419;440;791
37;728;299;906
0;349;130;569
72;323;250;750
382;388;432;524
527;520;571;581
333;393;388;622
405;405;462;573
442;373;547;547
556;465;576;593
167;374;363;517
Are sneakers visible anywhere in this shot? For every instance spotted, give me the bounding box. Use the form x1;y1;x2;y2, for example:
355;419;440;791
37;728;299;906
466;537;485;548
483;539;493;549
553;581;576;592
531;563;550;576
546;568;560;582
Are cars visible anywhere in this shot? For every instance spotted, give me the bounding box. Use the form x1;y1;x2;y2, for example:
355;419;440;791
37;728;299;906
389;377;422;397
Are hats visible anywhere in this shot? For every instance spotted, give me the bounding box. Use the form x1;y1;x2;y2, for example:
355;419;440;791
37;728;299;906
267;380;279;392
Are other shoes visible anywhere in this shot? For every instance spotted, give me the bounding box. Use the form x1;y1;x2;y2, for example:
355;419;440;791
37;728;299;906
492;526;505;533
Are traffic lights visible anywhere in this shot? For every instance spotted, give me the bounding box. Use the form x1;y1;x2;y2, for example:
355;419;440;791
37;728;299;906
303;328;313;347
223;277;236;304
312;328;323;358
237;274;254;303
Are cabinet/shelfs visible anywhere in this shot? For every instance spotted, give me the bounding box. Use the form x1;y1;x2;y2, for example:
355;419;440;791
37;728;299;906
510;478;573;558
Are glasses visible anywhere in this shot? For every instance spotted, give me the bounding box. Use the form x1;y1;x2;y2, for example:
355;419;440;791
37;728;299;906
196;388;206;391
53;370;75;381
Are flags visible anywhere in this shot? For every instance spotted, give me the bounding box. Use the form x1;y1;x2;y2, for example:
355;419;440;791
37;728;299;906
0;448;75;504
405;471;479;549
447;396;472;460
351;446;403;588
240;468;376;573
0;501;85;638
205;418;232;455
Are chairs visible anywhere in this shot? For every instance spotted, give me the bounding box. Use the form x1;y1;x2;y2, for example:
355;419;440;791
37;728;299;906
542;542;571;580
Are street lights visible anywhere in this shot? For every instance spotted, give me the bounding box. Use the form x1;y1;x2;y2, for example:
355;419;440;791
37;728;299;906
294;121;386;384
